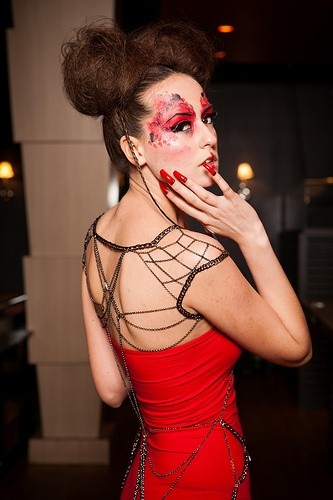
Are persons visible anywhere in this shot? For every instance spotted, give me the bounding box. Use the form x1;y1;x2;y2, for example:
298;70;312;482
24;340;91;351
59;21;314;500
